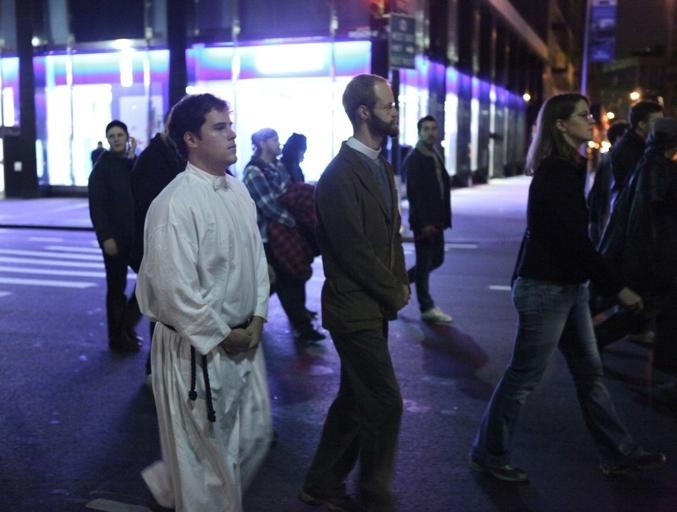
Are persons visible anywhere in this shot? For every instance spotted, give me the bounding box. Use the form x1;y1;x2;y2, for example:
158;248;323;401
401;116;453;325
91;140;106;165
471;92;668;484
128;107;189;389
242;128;325;343
296;73;411;511
89;120;142;353
134;92;276;511
590;102;677;377
283;132;318;320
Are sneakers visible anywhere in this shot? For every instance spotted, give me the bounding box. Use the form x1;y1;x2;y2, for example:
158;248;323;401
297;485;361;512
600;447;666;478
469;455;529;486
108;328;142;352
420;306;452;324
294;325;326;343
